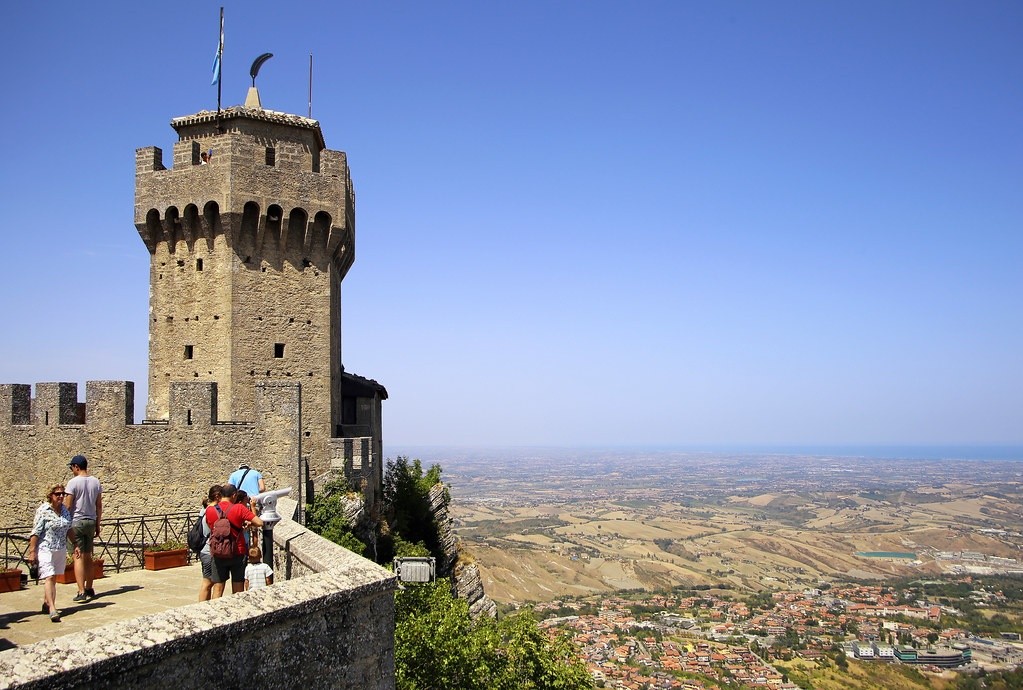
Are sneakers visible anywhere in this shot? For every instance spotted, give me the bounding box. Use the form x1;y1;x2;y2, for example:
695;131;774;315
72;588;95;603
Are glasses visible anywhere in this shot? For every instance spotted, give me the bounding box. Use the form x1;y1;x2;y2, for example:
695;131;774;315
52;491;66;497
69;466;73;471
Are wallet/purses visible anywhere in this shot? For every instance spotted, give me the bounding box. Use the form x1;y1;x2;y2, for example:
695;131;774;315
30;566;38;579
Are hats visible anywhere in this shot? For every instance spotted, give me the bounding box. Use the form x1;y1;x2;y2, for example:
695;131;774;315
66;455;88;470
238;463;249;469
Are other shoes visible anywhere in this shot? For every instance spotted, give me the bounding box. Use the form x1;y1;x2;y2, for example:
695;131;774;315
42;602;63;623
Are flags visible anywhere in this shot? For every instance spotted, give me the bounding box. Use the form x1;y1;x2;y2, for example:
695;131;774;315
211;16;224;85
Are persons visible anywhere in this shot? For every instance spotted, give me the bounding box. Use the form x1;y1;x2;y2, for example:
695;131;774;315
188;485;221;601
199;148;212;165
28;483;81;621
244;546;273;591
65;455;102;604
205;463;265;600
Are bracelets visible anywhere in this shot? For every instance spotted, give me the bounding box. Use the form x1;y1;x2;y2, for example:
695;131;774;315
72;541;78;544
29;547;36;553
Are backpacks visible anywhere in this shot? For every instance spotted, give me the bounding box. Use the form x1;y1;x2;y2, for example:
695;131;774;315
187;512;211;553
209;502;237;560
